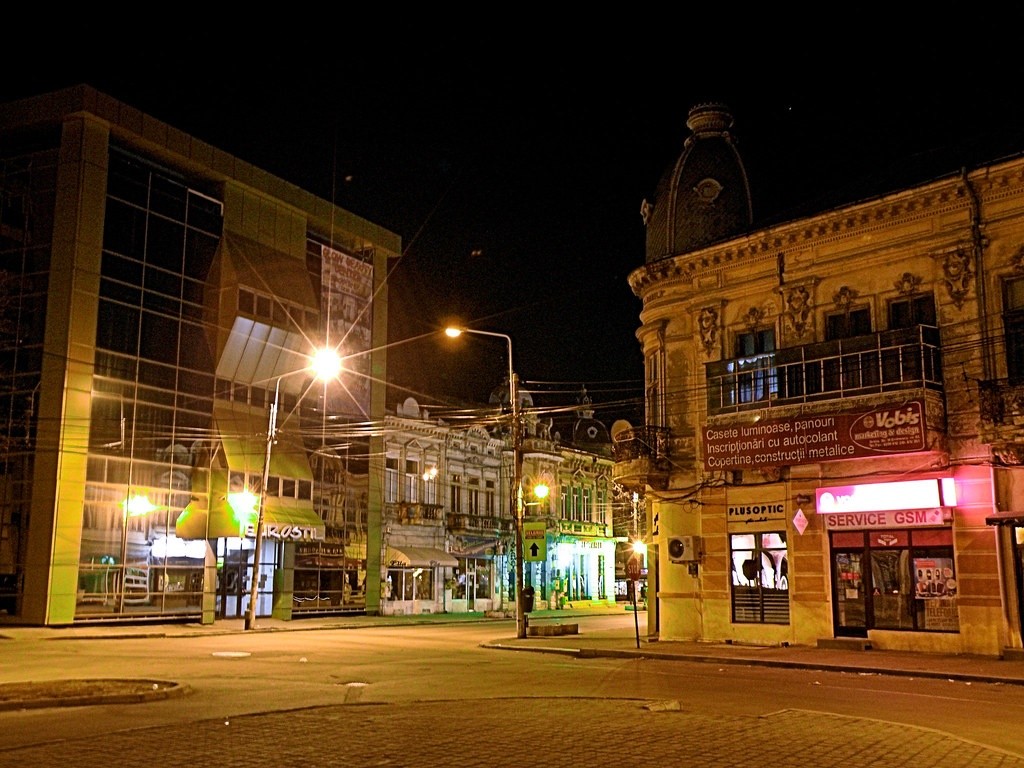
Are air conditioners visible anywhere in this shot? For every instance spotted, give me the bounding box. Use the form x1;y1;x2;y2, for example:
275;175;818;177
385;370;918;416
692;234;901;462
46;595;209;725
668;535;700;561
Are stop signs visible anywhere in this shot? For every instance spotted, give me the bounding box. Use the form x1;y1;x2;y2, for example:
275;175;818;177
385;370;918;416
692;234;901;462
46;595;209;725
625;558;641;581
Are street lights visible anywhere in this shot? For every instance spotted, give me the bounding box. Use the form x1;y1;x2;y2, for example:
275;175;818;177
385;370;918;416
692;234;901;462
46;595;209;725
245;345;346;630
445;324;530;640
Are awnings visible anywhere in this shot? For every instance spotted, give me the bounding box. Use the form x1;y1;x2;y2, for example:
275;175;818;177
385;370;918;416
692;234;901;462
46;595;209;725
384;546;458;569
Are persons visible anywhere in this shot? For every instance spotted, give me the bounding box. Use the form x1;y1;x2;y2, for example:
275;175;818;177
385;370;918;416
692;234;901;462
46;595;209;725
641;582;648;611
146;549;161;573
